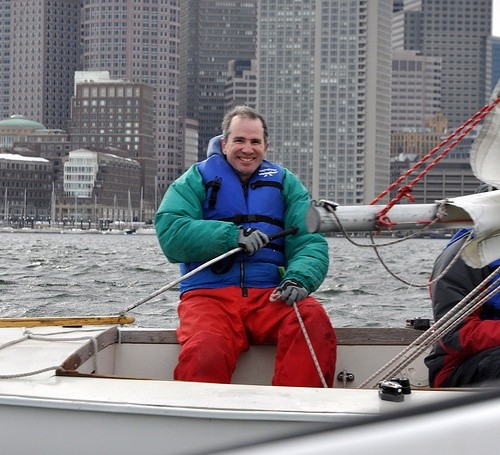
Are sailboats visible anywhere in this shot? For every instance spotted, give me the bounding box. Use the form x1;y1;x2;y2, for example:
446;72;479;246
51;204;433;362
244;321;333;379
0;174;165;236
0;92;499;454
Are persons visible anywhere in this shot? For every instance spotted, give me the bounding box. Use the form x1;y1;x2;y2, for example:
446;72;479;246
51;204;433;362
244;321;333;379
153;104;338;389
424;226;500;390
